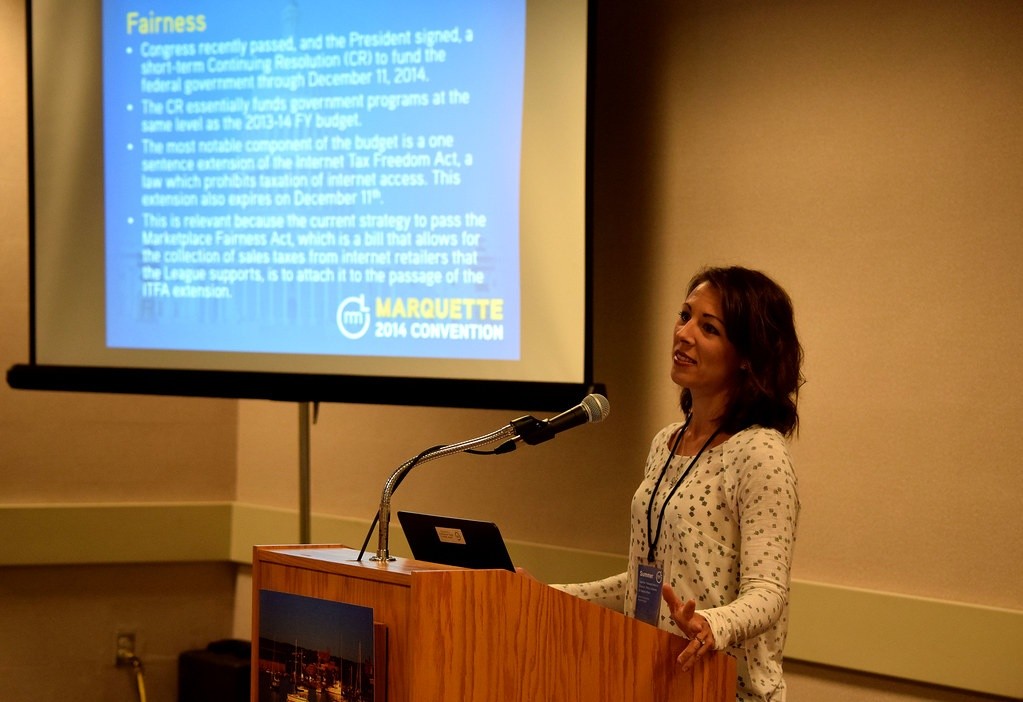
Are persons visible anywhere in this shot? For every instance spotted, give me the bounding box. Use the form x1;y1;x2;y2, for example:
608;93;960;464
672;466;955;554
515;265;787;702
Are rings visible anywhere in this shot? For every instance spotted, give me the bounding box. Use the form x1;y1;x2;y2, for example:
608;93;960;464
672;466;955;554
694;635;707;645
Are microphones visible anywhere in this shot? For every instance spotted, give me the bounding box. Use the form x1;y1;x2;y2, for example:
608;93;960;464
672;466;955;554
494;393;611;455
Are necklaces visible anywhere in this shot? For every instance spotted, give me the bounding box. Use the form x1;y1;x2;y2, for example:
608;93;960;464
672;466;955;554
668;421;720;488
